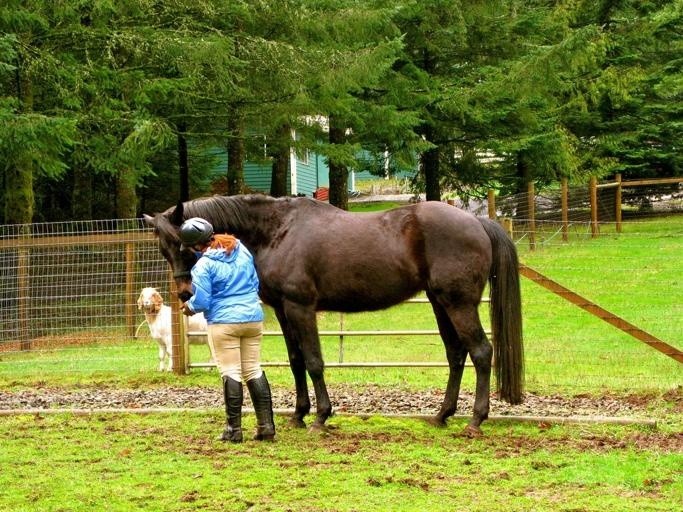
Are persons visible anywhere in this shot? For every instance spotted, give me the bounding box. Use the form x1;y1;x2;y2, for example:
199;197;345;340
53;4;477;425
177;217;276;443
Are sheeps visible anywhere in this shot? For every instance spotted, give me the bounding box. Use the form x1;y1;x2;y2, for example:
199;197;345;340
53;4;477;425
137;287;216;372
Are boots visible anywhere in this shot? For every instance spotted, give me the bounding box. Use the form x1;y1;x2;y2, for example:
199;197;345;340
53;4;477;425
221;376;243;441
247;370;275;440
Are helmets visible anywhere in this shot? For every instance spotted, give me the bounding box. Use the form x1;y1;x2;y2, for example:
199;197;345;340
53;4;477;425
180;218;214;252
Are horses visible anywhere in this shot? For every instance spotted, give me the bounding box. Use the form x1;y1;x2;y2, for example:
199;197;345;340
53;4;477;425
144;194;527;440
